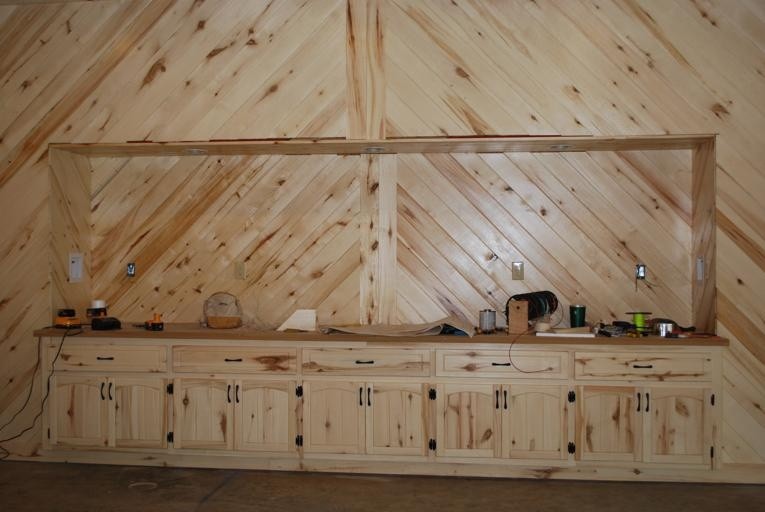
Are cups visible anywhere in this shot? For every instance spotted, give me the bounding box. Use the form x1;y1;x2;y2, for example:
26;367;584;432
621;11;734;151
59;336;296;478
570;304;586;328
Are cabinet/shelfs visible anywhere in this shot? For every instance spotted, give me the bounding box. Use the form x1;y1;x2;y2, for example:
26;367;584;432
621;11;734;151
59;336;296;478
300;332;434;475
575;337;731;481
36;327;169;466
434;336;574;480
169;331;299;470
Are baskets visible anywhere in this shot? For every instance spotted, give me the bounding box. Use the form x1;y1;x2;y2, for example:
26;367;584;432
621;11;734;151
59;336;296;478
203;291;242;329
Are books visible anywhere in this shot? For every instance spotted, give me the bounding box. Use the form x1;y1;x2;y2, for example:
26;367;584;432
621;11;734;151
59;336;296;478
536;326;596;339
